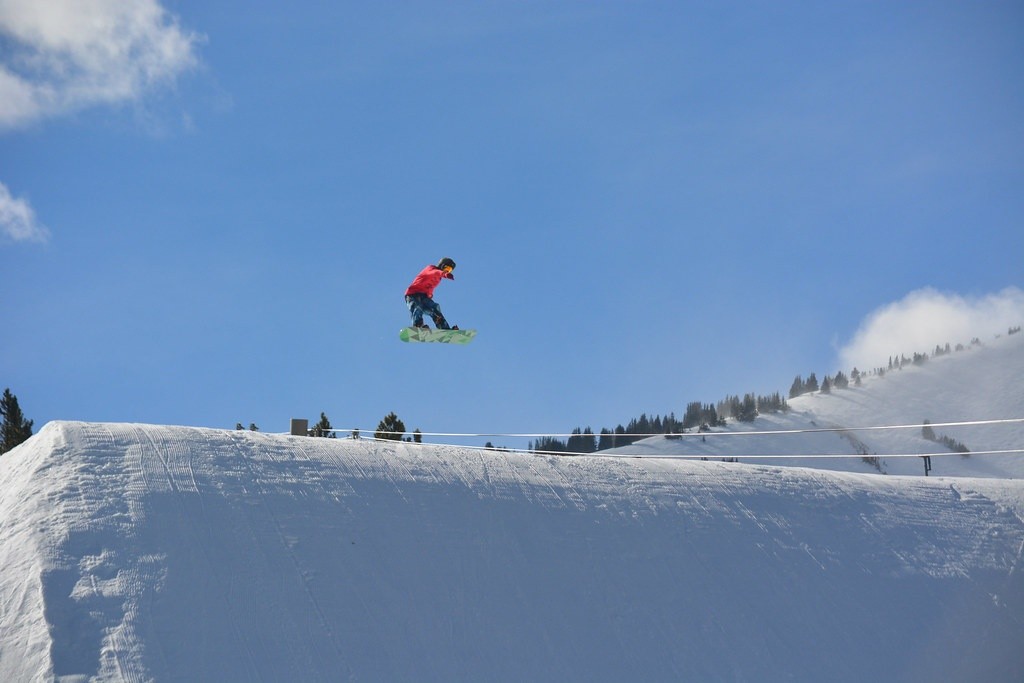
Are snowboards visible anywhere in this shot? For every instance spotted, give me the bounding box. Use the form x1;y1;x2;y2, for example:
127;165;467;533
398;326;478;344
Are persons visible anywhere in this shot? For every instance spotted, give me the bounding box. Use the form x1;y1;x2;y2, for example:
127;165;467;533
237;423;244;430
249;423;259;431
404;258;460;330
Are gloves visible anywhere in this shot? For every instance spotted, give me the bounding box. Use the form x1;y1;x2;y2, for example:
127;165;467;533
447;273;454;280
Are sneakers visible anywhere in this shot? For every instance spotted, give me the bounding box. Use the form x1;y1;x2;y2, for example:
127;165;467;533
452;325;459;330
416;323;429;329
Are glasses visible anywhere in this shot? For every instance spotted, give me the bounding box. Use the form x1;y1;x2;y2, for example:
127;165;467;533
443;266;452;271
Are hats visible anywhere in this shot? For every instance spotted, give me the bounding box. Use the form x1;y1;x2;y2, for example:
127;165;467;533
437;258;456;270
